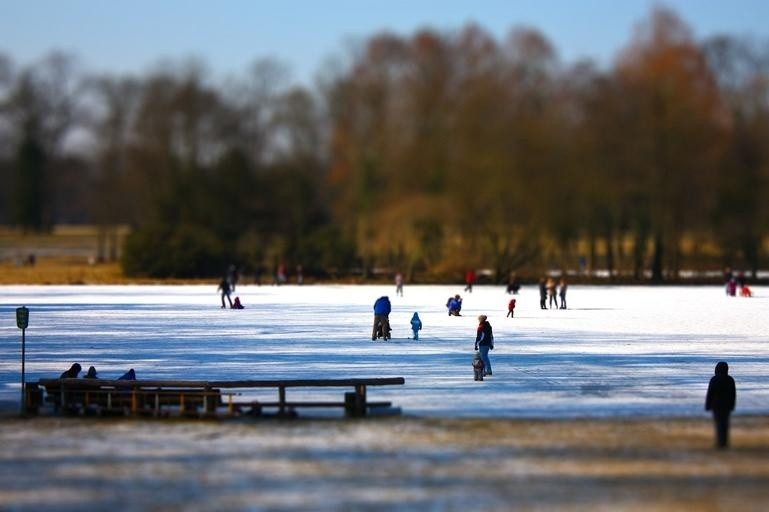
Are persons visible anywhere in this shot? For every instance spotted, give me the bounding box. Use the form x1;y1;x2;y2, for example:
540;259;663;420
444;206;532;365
83;366;98;378
704;362;736;449
394;272;403;296
448;294;463;317
725;278;736;295
472;352;484;381
559;278;568;309
118;368;138;379
216;276;233;308
739;282;751;297
371;296;391;341
410;312;422;340
474;314;494;375
506;298;516;317
465;269;473;292
59;361;81;378
547;276;558;308
539;277;548;310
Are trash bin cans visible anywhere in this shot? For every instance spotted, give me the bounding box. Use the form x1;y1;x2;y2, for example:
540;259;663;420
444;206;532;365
24;382;44;416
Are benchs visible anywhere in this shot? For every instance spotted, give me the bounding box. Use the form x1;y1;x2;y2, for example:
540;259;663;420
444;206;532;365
41;377;230;411
